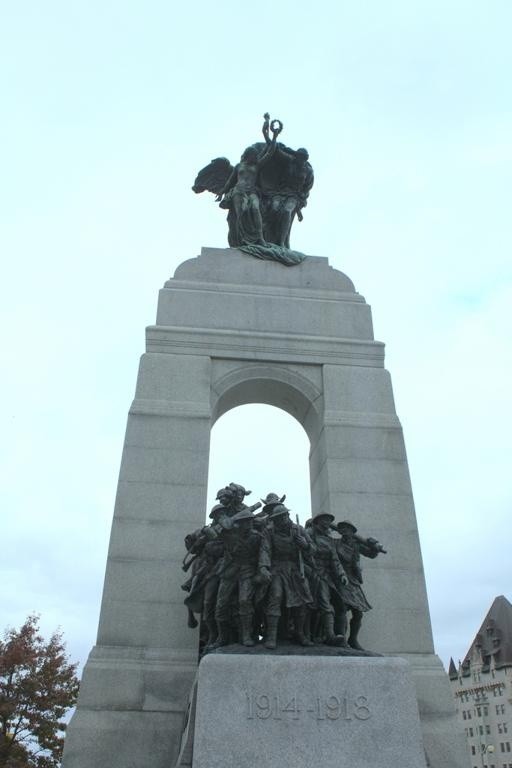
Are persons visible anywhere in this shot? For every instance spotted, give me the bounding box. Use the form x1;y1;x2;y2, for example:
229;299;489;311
214;120;279;249
180;482;383;656
261;112;313;249
